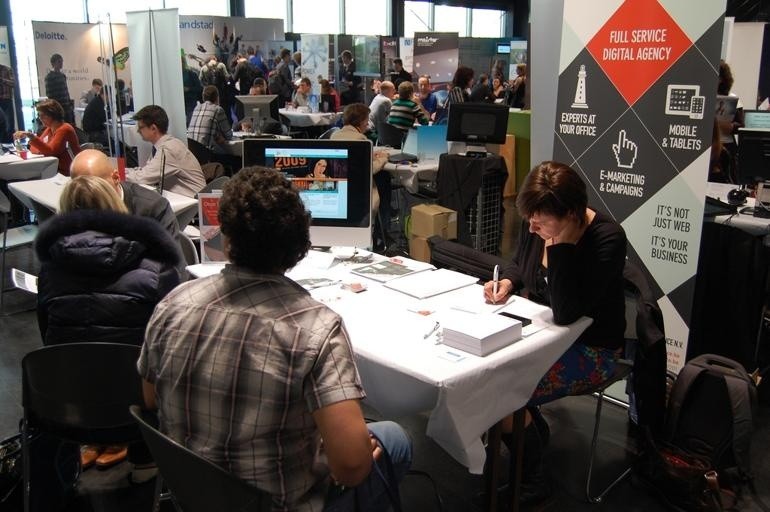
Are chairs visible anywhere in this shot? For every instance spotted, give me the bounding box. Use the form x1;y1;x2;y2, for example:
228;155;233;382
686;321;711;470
585;262;666;504
127;402;283;512
375;119;409;149
21;341;141;512
0;189;41;315
190;175;231;251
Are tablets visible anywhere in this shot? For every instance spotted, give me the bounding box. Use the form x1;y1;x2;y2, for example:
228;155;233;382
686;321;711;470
665;83;700;116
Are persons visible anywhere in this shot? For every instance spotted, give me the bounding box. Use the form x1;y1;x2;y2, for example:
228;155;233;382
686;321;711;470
0;64;16;113
179;45;529;153
114;106;208;202
483;161;627;489
82;76;136;155
44;54;75;131
29;148;186;491
328;103;390;239
9;98;80;228
136;167;412;511
709;61;747;182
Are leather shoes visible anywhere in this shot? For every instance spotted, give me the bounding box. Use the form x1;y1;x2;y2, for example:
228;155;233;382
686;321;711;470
81;446;160;484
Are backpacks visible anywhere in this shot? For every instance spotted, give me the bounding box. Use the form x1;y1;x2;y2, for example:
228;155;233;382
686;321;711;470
666;354;758;495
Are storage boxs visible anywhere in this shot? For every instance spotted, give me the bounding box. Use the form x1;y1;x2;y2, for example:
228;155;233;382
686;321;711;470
411;203;458;241
409;238;431;262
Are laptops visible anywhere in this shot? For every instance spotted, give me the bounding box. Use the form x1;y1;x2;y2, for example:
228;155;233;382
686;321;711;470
66;140;75;161
151;147;165;195
319;94;336;112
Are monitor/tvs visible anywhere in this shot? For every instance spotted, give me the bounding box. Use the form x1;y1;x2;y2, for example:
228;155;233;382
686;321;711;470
732;109;770;147
234;95;283;140
497;43;511;54
241;137;373;249
737;128;770;219
445;102;510;157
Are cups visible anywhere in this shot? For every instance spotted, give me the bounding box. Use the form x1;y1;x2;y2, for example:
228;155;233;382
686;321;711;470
20;151;27;160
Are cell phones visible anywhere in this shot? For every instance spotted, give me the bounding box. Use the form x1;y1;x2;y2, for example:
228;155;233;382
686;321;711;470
689;95;706;120
498;311;532;328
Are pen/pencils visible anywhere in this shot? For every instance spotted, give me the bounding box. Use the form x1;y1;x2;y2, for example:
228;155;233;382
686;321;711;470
493;264;500;304
424;322;440;339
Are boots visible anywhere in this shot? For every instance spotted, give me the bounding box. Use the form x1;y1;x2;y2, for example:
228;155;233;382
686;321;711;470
495;422;552;510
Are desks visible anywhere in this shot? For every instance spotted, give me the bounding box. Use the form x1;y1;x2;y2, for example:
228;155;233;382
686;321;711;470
279;107;342;129
103;116;137;147
698;180;770;360
0;144;59;179
372;144;442;189
7;172;201;236
228;131;289;157
182;242;597;511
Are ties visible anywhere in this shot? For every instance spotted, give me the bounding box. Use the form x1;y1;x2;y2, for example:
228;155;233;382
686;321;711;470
152;145;157;159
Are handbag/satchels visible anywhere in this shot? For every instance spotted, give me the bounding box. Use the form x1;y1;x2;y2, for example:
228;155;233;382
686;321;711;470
647;440;721;512
